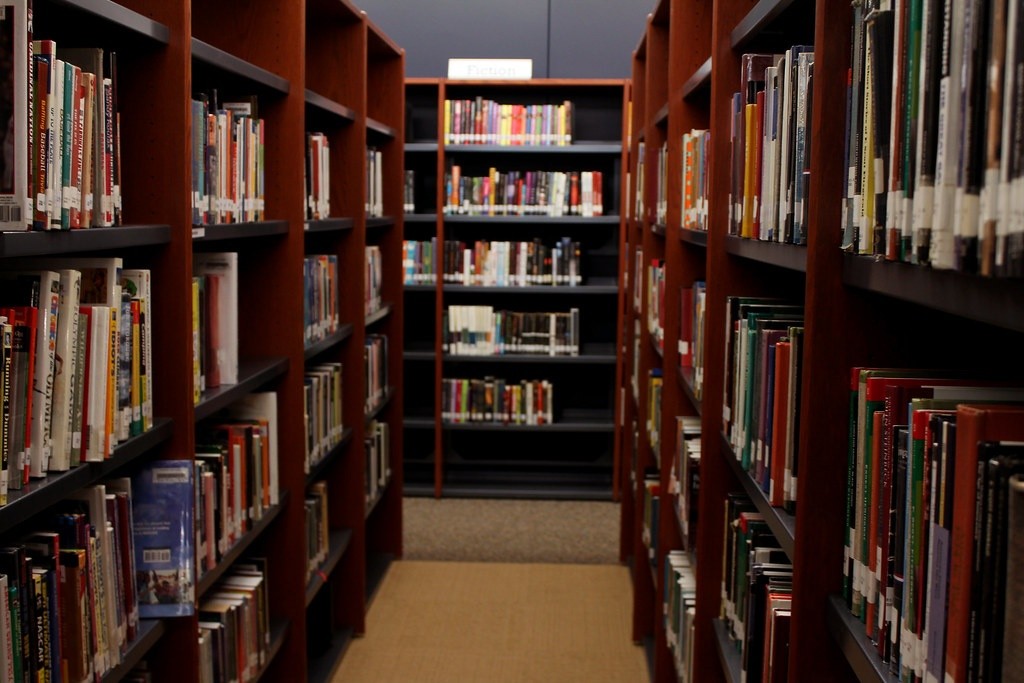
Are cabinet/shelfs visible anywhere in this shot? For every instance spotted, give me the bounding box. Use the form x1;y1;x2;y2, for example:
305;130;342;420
0;0;1024;683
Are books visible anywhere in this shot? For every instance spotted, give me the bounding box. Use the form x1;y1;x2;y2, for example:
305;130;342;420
400;97;603;427
620;0;1024;683
0;0;392;683
